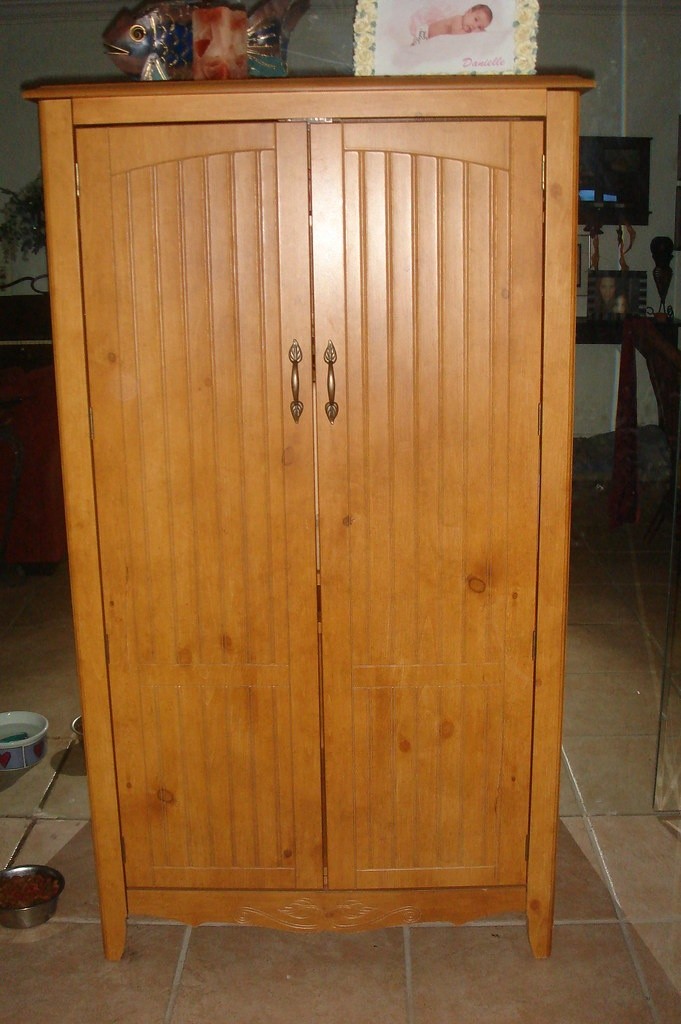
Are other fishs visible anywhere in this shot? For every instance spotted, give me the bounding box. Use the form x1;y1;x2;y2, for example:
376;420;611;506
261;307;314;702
102;0;311;81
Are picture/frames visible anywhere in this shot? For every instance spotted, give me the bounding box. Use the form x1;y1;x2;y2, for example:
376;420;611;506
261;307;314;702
351;0;541;77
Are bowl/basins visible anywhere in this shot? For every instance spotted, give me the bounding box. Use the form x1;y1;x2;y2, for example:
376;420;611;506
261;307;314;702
0;865;65;930
0;711;50;772
71;715;84;746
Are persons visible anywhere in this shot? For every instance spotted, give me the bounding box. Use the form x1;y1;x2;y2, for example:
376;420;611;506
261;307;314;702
408;5;494;43
590;277;629;317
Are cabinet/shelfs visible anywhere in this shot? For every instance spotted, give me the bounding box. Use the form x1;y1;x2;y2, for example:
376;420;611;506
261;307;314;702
23;76;595;963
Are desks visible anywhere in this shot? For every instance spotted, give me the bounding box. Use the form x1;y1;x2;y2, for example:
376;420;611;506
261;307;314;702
575;315;681;525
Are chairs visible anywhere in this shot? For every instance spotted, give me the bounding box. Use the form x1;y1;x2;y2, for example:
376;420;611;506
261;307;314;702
623;317;681;548
